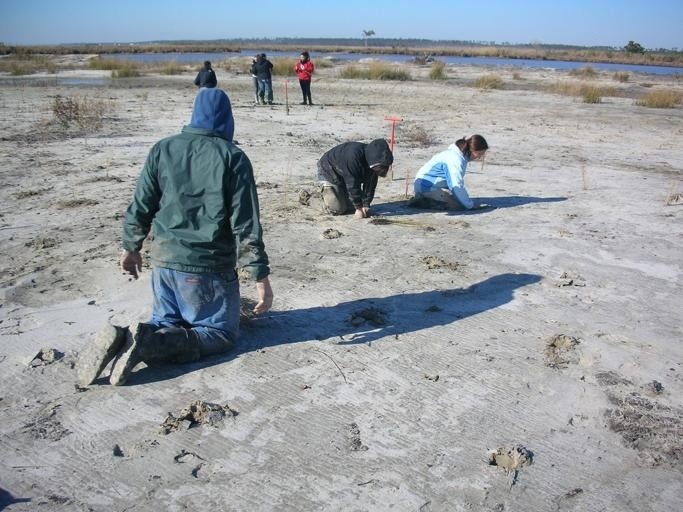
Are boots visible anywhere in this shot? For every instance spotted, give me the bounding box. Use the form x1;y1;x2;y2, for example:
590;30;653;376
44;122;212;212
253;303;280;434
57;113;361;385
406;194;448;210
77;325;129;385
109;323;196;386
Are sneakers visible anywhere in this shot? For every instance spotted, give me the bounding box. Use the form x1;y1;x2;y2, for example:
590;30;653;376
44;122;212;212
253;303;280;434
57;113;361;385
301;102;312;105
256;100;272;104
299;189;311;205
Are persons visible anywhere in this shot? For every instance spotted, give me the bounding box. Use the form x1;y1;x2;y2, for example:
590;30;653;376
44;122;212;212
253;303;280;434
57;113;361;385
71;88;274;387
406;134;489;211
294;138;393;219
248;55;260;104
251;53;275;105
193;61;216;90
293;51;315;106
